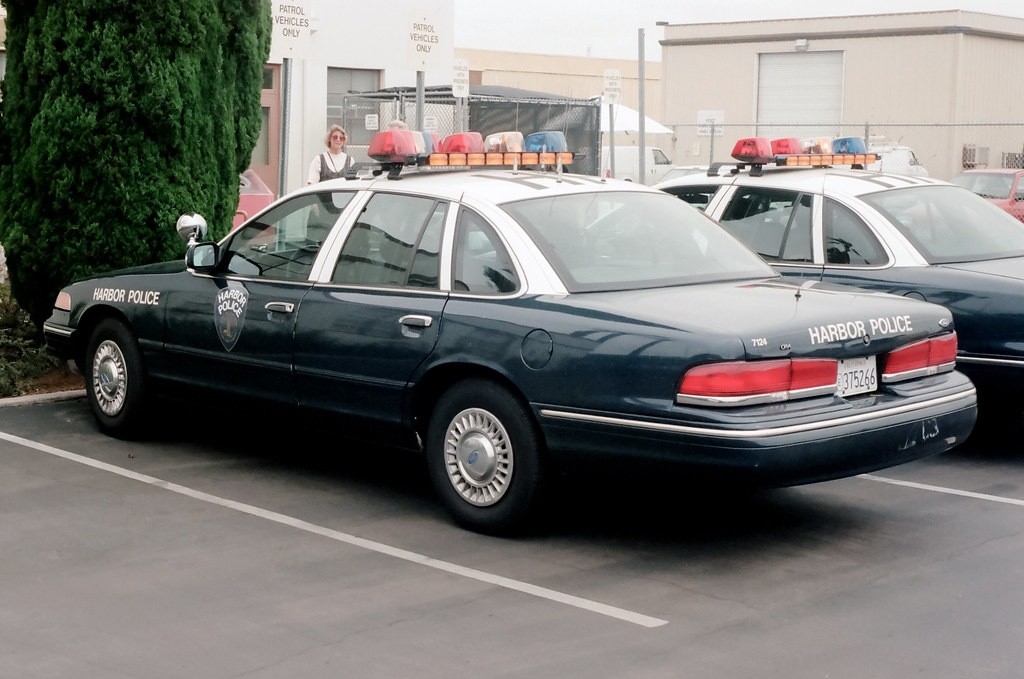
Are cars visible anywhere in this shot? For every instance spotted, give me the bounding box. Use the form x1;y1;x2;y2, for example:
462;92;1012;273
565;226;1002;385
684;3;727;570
41;130;981;539
946;165;1024;224
579;132;1023;445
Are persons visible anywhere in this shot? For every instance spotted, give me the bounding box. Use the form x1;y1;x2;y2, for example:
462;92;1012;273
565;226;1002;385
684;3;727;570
387;120;408;131
307;124;355;241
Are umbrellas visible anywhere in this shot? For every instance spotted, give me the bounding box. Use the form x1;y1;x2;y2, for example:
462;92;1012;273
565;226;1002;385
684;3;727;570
539;91;675;134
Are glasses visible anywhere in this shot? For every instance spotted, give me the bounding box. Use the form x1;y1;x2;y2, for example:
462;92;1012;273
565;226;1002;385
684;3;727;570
331;135;346;142
